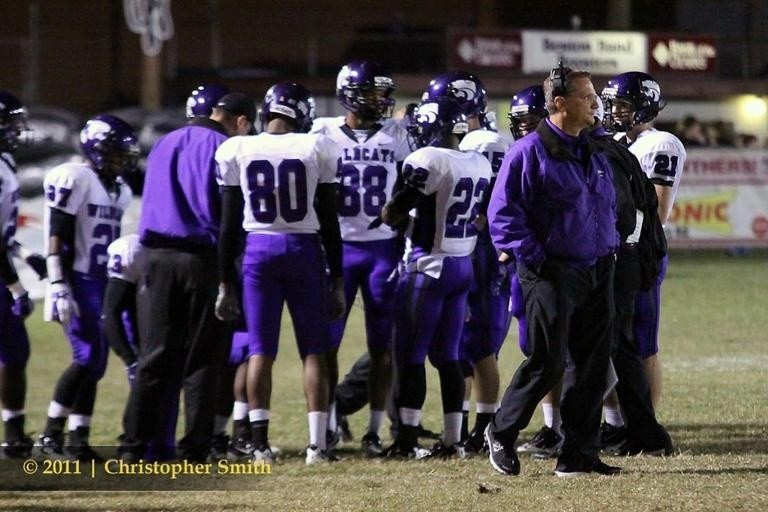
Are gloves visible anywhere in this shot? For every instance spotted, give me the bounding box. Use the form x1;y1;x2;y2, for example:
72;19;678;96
5;282;34;317
45;283;82;325
214;284;241;322
326;284;348;321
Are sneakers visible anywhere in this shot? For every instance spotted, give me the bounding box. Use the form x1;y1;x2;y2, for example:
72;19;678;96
209;435;252;465
375;443;424;458
5;436;34;457
65;439;105;463
333;396;353;443
109;450;138;474
603;424;673;456
137;453;177;467
29;434;76;463
360;434;381;454
553;454;621;480
516;426;565;458
594;422;626;449
305;447;347;467
418;443;467;463
253;448;275;469
484;423;520;475
461;424;487;452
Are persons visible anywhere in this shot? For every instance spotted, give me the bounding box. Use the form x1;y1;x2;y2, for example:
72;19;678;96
1;93;34;457
214;79;348;465
309;58;418;455
383;96;492;458
421;70;512;458
507;86;627;455
483;60;624;479
100;233;138;383
186;81;257;463
600;72;687;462
117;91;257;470
44;113;139;468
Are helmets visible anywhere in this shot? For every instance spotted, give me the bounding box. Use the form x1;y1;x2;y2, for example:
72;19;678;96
507;85;549;142
403;96;461;140
372;76;398;120
0;90;33;147
336;62;382;122
255;83;316;133
78;115;131;178
598;72;667;132
413;70;488;119
185;83;231;119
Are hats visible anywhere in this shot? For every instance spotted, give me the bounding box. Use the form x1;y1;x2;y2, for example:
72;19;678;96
216;92;258;135
